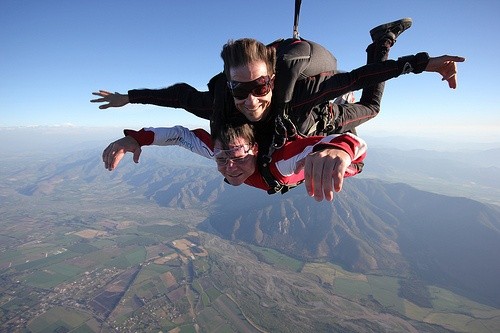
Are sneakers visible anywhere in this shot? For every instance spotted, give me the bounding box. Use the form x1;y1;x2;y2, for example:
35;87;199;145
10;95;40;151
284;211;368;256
369;19;412;45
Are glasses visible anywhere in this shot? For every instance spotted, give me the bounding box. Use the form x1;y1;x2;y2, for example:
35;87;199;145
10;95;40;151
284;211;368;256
227;74;275;99
214;147;254;165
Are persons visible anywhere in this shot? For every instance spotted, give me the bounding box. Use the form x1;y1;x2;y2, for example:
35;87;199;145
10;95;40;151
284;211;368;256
101;92;366;202
90;18;466;134
